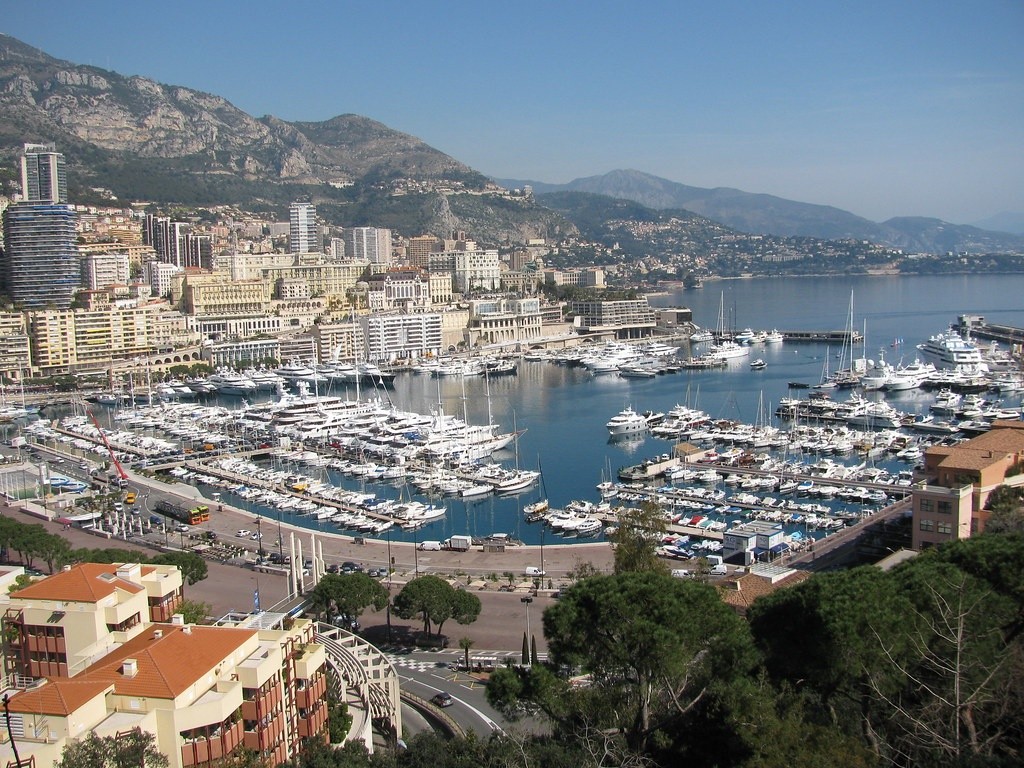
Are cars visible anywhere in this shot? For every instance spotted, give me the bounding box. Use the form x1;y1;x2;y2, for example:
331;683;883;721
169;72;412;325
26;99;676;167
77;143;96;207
205;530;217;540
148;514;163;526
56;456;65;464
433;691;453;708
250;533;264;540
236;529;250;538
79;463;88;469
132;506;141;514
177;524;189;533
126;492;136;504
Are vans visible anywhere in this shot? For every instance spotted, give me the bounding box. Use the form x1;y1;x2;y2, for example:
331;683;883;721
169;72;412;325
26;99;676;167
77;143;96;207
418;540;441;551
526;566;546;578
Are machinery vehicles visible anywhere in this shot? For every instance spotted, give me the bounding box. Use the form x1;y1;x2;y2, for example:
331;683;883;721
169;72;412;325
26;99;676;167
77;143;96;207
84;404;131;483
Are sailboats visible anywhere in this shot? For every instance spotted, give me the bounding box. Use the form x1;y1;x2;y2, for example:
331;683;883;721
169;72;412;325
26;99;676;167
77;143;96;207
11;285;1023;575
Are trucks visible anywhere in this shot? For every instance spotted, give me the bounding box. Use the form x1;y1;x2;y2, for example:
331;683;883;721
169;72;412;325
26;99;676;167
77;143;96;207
443;534;472;552
